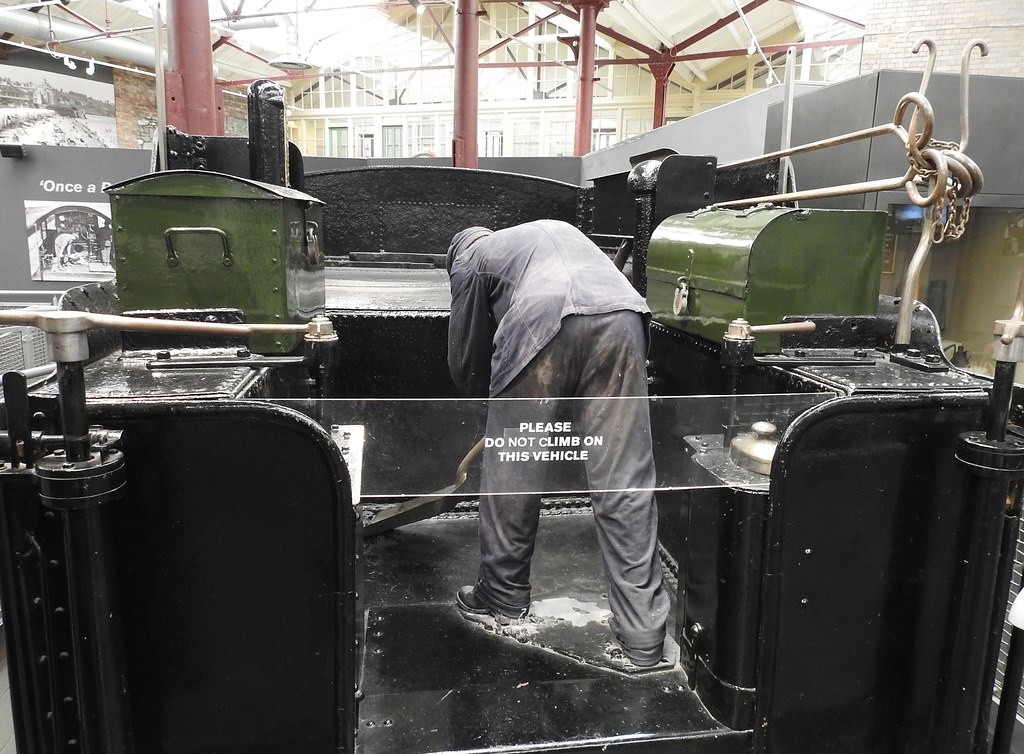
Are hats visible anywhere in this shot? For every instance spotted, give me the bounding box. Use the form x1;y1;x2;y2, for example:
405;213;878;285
445;226;493;277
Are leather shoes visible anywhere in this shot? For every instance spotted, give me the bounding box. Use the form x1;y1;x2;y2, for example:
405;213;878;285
616;638;664;667
456;586;529;624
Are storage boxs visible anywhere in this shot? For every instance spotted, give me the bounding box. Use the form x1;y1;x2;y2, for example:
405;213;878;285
106;171;326;358
646;207;897;355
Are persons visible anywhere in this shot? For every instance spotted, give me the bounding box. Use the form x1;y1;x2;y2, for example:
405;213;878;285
99;220;112;263
55;233;79;271
447;218;670;668
